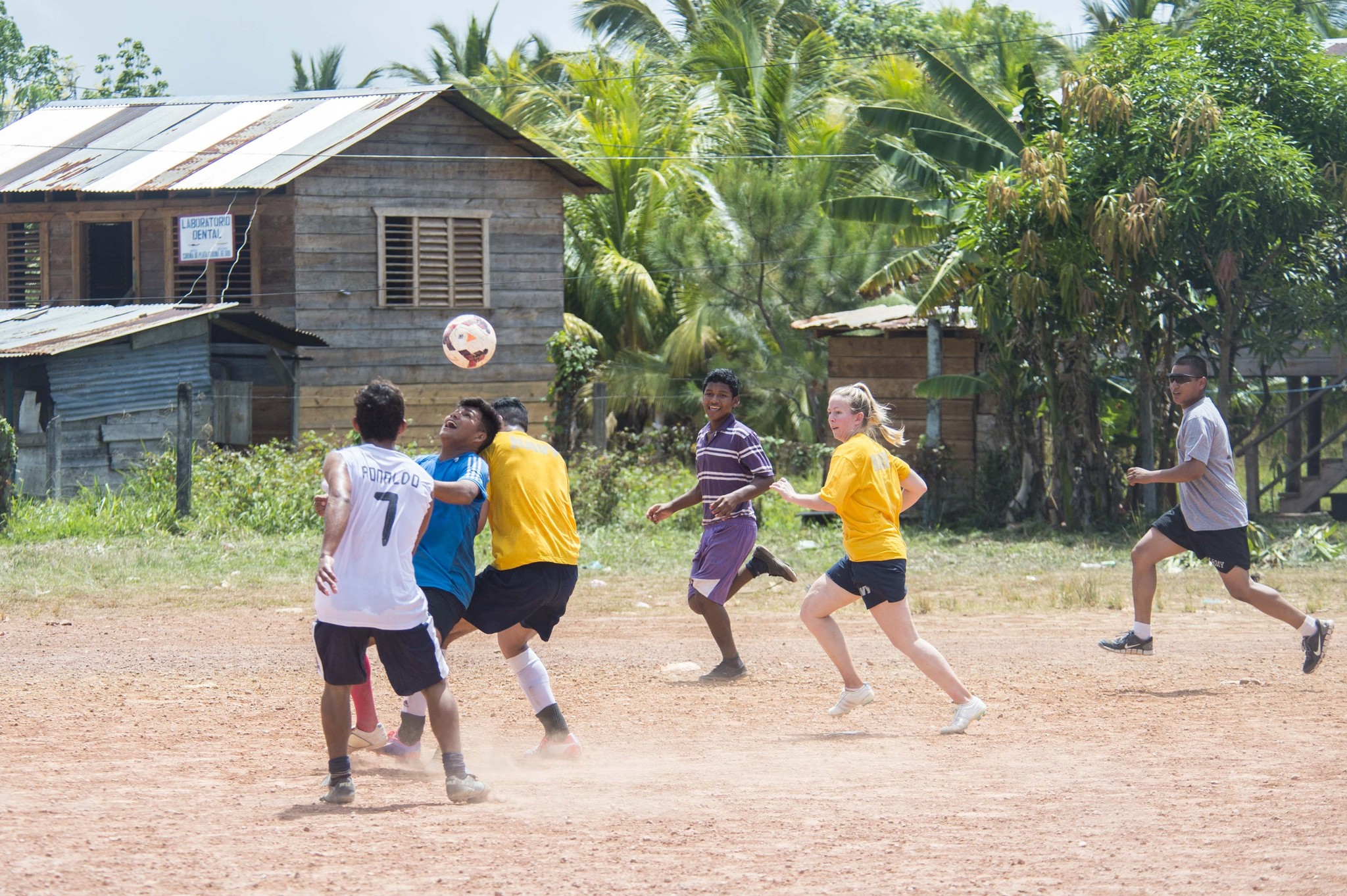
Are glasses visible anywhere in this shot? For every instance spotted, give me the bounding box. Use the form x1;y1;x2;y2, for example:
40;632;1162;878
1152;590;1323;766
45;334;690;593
1169;372;1202;383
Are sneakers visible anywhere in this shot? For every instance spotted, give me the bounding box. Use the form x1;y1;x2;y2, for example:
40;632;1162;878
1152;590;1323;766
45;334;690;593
939;697;987;734
828;683;876;718
446;774;490;802
372;730;422;762
1301;620;1334;673
752;545;797;583
1098;630;1154;655
324;722;389;755
524;733;582;760
320;774;355;802
698;659;749;681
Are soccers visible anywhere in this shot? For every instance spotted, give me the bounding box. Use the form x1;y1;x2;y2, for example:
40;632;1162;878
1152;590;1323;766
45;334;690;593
441;314;496;369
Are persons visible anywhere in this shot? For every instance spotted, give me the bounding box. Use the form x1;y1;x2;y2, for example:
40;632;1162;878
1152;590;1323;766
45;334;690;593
316;380;493;804
1098;356;1334;674
345;397;503;754
646;369;797;683
370;395;580;766
769;382;987;735
